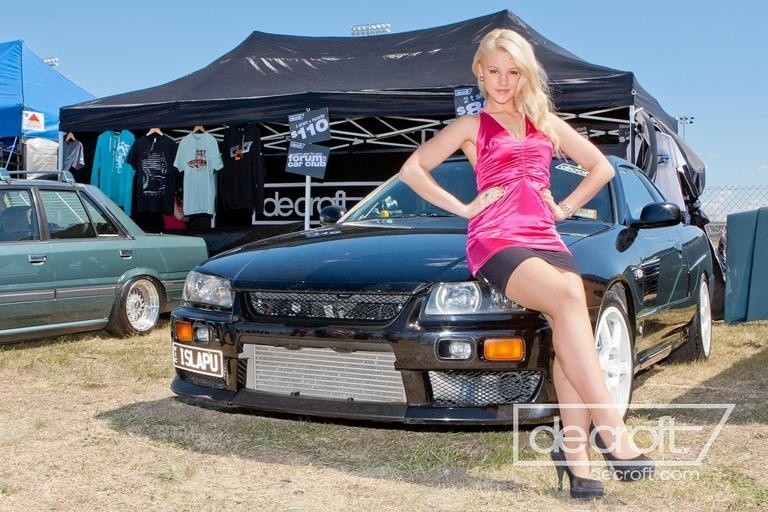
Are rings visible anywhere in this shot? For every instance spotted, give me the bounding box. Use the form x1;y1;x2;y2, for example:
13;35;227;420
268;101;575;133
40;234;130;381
484;191;491;198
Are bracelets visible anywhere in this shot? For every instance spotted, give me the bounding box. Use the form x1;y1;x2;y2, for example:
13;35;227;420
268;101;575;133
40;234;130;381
558;200;576;220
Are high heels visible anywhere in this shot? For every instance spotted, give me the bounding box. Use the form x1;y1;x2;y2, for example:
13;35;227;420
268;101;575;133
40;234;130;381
589;419;655;481
551;441;604;500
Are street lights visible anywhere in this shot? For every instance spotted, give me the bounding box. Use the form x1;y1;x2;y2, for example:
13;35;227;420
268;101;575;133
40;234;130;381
350;23;390;35
45;56;62;67
677;114;694;145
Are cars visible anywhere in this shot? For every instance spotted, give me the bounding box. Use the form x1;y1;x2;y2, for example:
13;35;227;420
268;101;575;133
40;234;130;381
168;153;717;427
1;172;208;342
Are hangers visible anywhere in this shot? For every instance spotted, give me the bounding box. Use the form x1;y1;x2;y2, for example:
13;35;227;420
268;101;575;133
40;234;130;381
190;125;206;134
64;131;75;142
145;127;164;137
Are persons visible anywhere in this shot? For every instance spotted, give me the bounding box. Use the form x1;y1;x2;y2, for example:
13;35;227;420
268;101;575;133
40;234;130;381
398;27;656;499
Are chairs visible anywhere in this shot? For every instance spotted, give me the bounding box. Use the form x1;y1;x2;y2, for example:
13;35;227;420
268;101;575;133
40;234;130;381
0;206;34;241
